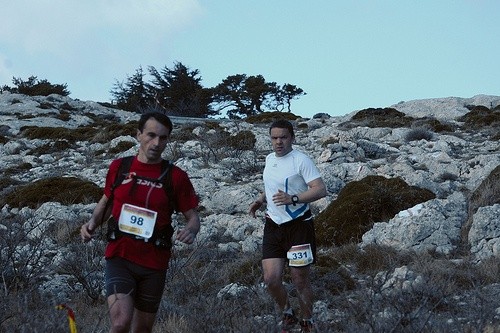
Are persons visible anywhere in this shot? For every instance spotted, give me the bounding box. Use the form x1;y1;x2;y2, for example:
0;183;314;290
80;109;202;333
248;119;328;333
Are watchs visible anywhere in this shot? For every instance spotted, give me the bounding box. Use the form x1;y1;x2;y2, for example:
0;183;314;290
290;194;299;206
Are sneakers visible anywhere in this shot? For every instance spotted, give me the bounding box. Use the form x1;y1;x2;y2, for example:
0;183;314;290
299;318;313;332
283;310;298;330
